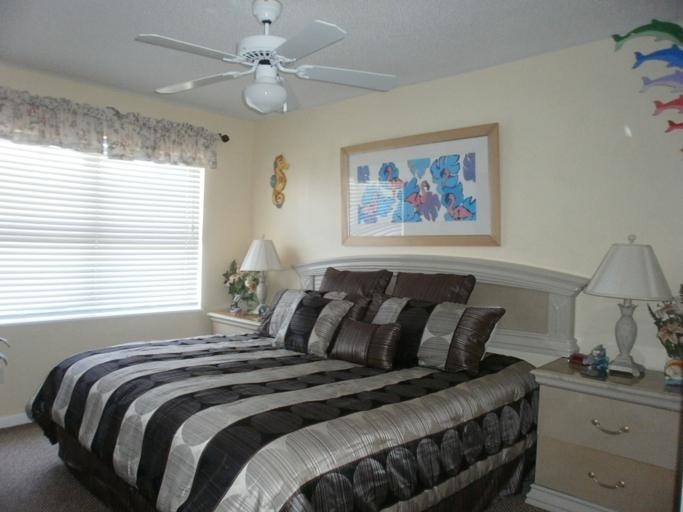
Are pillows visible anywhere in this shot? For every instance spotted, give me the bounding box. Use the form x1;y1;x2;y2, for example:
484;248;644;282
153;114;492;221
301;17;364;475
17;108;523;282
393;271;476;304
319;267;392;300
364;292;505;376
258;289;351;337
328;316;400;368
272;292;353;359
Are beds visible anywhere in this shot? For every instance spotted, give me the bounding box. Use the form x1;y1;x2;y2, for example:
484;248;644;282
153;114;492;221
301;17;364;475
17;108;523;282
24;253;587;512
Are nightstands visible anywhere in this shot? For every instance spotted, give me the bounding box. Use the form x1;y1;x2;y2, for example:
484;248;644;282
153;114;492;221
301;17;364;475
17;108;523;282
209;311;259;335
524;356;682;511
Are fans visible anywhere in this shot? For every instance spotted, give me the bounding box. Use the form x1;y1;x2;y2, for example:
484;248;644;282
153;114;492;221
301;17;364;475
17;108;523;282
134;0;398;95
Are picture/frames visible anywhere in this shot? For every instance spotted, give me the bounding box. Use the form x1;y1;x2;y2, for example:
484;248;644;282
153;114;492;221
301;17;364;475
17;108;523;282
340;123;501;249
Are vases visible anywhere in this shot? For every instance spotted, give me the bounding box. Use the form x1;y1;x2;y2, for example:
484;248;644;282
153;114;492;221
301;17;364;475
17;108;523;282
230;300;240;312
664;361;683;385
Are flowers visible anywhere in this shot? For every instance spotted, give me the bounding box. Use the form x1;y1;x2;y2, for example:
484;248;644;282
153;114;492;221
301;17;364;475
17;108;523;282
645;298;681;358
223;262;259;304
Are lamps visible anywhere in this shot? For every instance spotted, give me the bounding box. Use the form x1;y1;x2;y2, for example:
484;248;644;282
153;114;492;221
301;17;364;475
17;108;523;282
582;232;669;378
242;239;285;315
244;67;287;112
